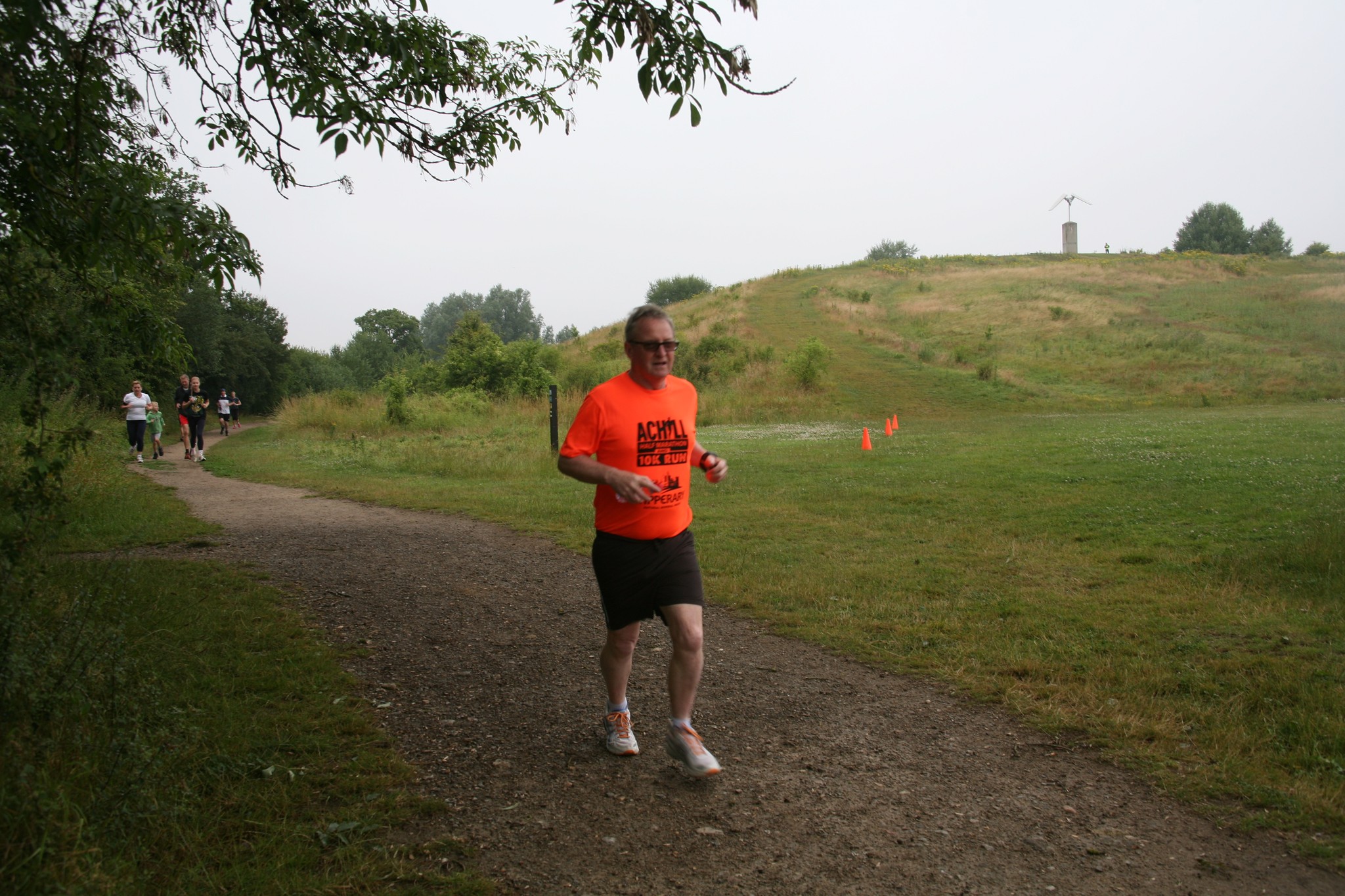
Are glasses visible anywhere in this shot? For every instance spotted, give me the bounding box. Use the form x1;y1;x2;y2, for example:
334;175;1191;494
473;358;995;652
627;340;680;351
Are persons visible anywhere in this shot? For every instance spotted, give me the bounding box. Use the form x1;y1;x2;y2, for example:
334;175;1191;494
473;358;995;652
215;389;234;436
174;375;209;462
120;381;153;463
229;392;242;429
554;302;728;777
146;402;167;460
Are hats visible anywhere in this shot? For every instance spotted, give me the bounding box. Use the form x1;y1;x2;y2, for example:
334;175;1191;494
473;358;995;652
220;388;225;391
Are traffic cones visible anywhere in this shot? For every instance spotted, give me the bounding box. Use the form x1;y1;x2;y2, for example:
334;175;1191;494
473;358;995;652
892;414;899;430
862;427;873;450
885;418;893;437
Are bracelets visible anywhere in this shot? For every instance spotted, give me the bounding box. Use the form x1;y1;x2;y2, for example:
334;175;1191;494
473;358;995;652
699;451;718;473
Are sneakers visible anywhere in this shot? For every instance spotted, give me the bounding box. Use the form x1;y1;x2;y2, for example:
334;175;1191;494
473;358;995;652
153;453;158;460
198;455;206;461
185;453;191;460
220;426;225;434
238;423;241;428
226;430;229;436
130;443;138;455
158;447;164;456
666;717;721;778
190;451;197;462
137;457;144;463
233;426;236;429
603;703;639;755
